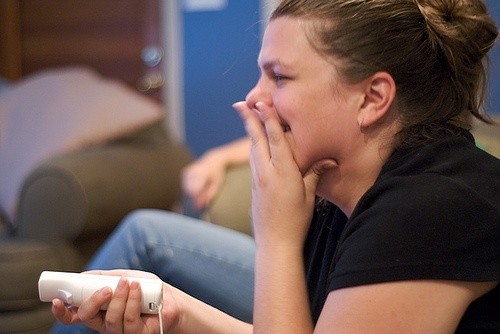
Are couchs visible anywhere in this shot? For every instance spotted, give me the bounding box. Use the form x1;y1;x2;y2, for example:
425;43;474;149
1;64;197;330
185;113;500;236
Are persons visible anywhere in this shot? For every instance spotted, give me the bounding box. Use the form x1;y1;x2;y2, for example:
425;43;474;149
51;1;500;334
50;134;256;334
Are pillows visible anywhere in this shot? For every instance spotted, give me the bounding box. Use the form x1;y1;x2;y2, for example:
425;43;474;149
1;66;169;229
